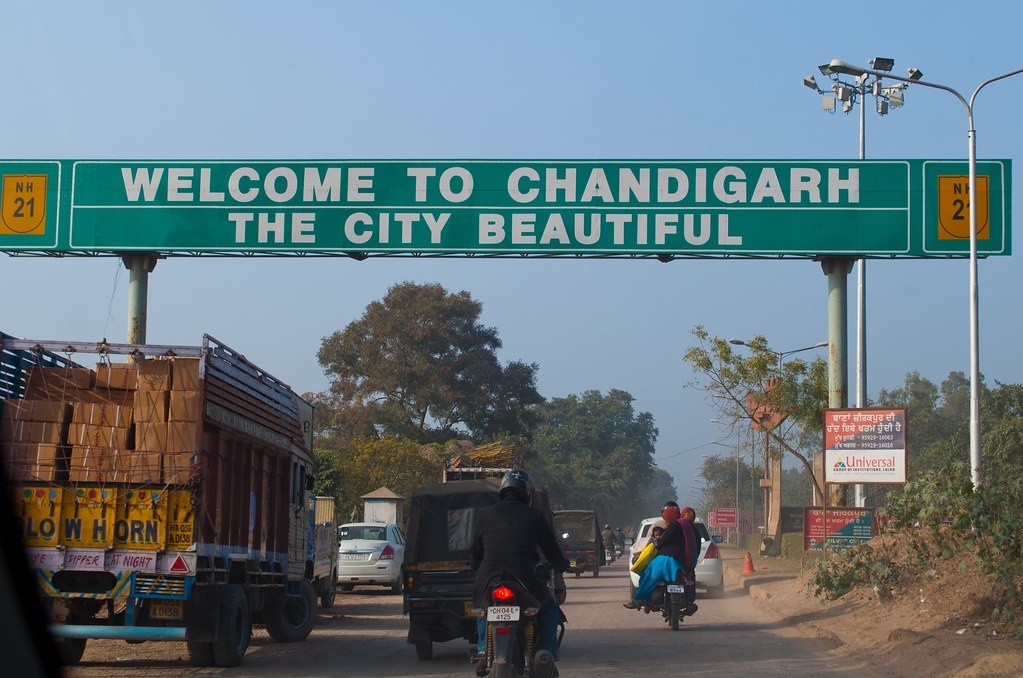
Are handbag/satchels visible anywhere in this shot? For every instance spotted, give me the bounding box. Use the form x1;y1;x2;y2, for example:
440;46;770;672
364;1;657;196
631;542;658;576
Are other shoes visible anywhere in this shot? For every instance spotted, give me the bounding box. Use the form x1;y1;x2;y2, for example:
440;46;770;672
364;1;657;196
644;599;651;613
686;603;698;616
475;655;486;675
623;599;642;611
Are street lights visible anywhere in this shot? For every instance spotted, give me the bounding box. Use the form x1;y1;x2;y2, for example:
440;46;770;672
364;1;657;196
826;58;1022;491
687;393;758;548
801;58;925;500
731;338;830;467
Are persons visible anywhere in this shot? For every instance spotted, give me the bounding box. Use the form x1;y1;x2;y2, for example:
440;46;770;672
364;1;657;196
614;527;626;552
628;528;636;538
475;468;571;678
623;501;702;616
601;524;618;549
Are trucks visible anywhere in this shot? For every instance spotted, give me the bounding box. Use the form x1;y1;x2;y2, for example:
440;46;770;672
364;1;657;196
313;494;341;607
445;468;513;480
1;331;321;668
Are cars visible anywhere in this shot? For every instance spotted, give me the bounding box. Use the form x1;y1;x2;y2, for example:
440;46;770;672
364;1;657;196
624;515;725;599
338;521;406;595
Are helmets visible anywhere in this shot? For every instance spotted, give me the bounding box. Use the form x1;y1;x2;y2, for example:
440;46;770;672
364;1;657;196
499;469;535;501
605;524;610;528
662;502;680;522
616;528;621;531
682;507;695;522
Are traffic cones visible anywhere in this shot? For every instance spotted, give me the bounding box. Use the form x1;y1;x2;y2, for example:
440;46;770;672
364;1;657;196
740;552;757;575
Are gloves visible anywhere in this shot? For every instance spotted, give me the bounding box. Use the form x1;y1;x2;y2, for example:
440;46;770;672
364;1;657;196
554;559;570;572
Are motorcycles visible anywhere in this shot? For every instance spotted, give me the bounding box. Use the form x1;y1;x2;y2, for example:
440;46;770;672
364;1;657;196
480;579;540;678
399;480;552;662
605;545;624;567
655;573;700;629
553;509;602;580
